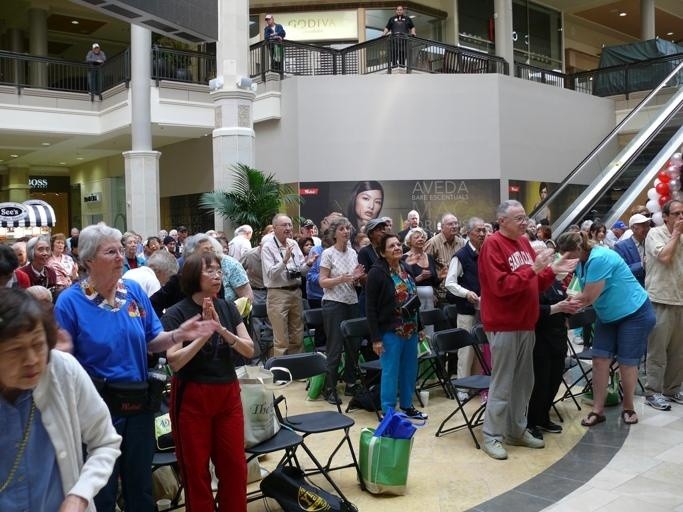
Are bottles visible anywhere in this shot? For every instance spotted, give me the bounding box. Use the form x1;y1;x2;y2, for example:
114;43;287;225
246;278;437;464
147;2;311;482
147;356;165;411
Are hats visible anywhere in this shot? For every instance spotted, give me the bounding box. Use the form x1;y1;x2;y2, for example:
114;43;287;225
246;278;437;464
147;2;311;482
629;214;652;228
613;221;628;229
365;218;385;234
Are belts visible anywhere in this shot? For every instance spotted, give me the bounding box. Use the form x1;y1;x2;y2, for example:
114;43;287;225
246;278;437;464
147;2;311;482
252;287;266;290
281;286;298;290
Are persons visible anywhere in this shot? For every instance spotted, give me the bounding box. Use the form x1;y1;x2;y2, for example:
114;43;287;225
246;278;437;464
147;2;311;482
85;43;106;95
347;181;384;248
2;200;683;511
381;4;416;68
533;182;551;228
264;14;285;74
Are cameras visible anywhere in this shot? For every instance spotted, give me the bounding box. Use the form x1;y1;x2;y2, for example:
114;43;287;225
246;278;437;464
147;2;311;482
287;269;300;279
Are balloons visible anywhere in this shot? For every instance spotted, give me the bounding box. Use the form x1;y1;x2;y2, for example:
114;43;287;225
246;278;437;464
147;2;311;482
646;152;683;226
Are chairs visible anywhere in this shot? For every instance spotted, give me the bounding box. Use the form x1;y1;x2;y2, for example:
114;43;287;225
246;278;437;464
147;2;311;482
261;352;362;477
431;328;493;449
152;451;218;512
214;426;304;512
252;303;649;424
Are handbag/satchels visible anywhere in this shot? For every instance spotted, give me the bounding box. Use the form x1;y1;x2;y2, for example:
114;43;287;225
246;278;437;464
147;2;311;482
103;381;149;417
359;407;416;496
581;372;620;406
401;296;420;318
260;466;356;511
306;349;382;411
235;364;281;449
155;390;175;453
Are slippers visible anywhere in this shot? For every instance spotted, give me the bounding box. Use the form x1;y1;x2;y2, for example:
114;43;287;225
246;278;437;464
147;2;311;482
621;410;637;424
581;412;605;425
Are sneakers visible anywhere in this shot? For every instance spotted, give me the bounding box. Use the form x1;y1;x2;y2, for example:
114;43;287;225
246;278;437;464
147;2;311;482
482;422;561;459
455;393;467;402
646;393;670;410
668;393;683;404
400;405;428;419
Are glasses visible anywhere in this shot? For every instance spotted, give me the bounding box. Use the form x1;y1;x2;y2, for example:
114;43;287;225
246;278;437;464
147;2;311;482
201;270;222;279
95;247;124;256
